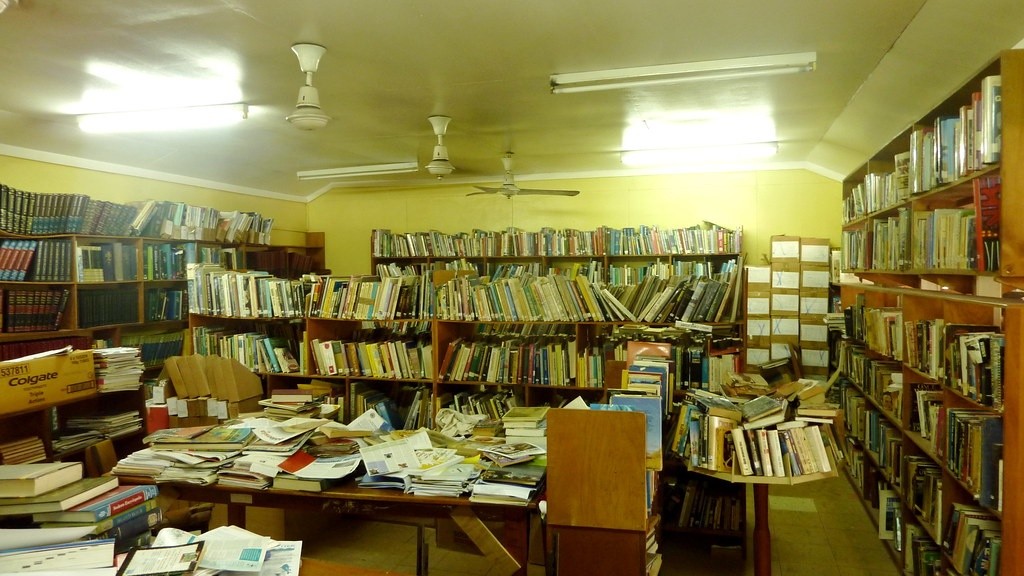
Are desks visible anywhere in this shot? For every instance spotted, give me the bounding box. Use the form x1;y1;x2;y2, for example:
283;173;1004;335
688;464;831;576
101;470;547;576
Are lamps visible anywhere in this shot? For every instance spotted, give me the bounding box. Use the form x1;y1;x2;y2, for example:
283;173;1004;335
297;160;418;182
549;49;817;95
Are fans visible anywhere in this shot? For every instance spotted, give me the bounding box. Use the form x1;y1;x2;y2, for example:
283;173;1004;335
198;42;580;198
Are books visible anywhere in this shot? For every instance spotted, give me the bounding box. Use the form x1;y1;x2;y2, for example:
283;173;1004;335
0;75;1024;576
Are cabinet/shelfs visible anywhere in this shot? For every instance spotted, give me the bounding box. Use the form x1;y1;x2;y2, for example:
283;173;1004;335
0;230;325;350
188;48;1024;576
0;384;151;477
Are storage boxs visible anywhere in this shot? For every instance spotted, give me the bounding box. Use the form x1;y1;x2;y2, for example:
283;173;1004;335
800;265;829;293
770;341;800;360
747;291;770;317
800;348;830;376
743;251;772;292
799;292;829;319
770;234;800;263
771;262;800;290
0;349;98;420
799;319;829;349
770;288;800;316
746;344;771;368
162;353;265;428
800;237;831;266
771;315;799;342
434;516;546;567
747;316;772;344
